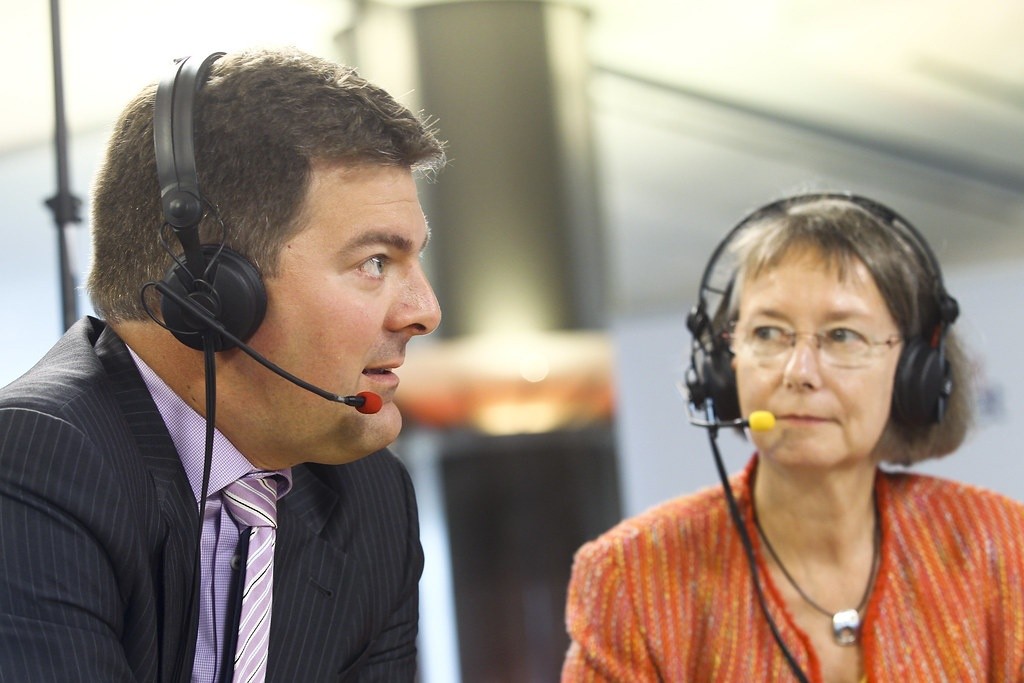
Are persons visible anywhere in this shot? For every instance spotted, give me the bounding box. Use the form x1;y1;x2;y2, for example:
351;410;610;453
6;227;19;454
553;197;1024;683
0;46;451;683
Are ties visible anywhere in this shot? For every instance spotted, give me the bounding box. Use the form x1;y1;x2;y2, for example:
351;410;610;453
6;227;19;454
219;475;278;683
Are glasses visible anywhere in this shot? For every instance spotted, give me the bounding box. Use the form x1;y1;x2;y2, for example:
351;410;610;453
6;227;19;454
722;320;905;369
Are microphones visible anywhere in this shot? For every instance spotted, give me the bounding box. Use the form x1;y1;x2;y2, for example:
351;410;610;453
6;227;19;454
685;399;775;431
143;278;382;417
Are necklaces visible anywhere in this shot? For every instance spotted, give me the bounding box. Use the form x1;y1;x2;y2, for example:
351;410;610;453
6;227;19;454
743;494;880;648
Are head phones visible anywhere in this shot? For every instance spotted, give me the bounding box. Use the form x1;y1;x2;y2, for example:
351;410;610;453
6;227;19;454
140;53;267;352
682;191;958;432
